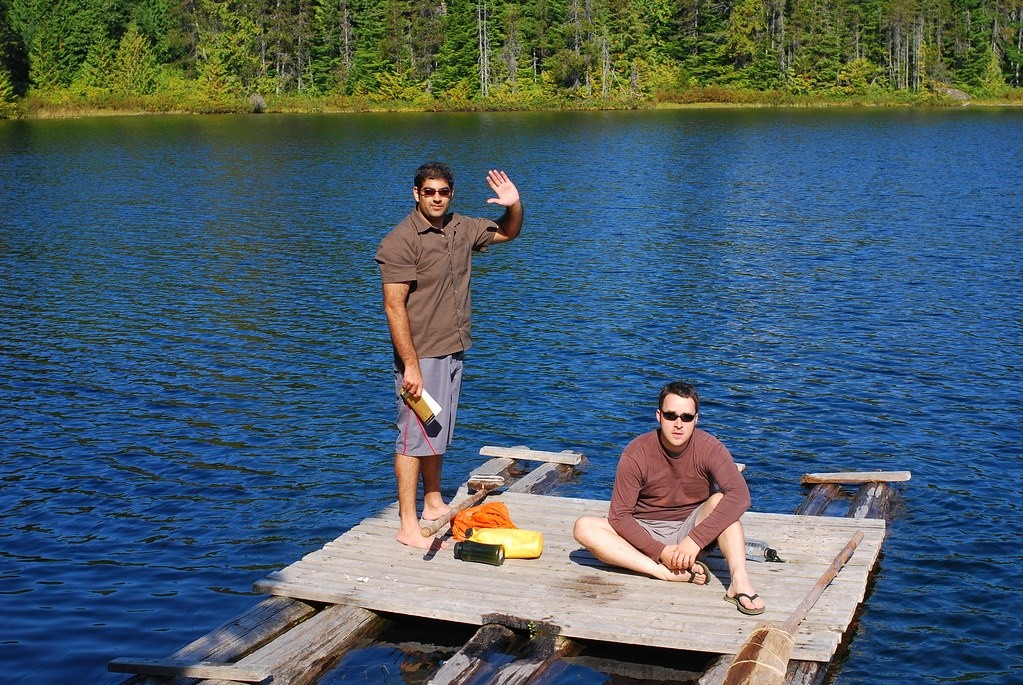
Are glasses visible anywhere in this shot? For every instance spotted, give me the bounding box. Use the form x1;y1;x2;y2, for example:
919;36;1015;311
661;409;697;422
418;187;452;197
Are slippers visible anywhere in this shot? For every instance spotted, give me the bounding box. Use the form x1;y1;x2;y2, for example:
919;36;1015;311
723;593;765;614
685;561;711;584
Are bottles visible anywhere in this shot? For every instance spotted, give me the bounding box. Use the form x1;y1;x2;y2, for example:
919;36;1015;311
465;527;543;559
454;540;505;566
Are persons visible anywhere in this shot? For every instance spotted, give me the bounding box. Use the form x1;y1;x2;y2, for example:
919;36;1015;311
374;161;524;548
574;380;767;616
